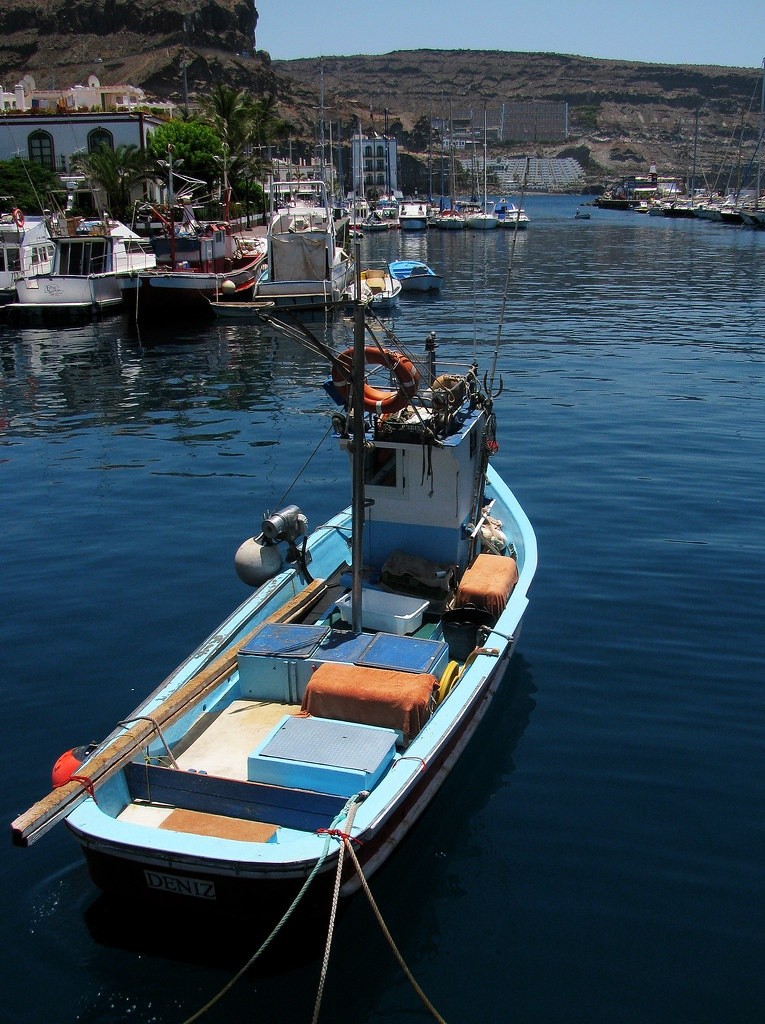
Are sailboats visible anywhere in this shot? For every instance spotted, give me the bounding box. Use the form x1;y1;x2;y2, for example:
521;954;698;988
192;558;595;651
0;62;531;319
594;59;765;229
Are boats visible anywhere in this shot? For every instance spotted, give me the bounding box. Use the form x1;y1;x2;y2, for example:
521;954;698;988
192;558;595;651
60;241;539;927
575;213;591;219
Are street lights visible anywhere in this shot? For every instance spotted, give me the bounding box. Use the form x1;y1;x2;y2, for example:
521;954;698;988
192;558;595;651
212;148;238;188
155;143;185;209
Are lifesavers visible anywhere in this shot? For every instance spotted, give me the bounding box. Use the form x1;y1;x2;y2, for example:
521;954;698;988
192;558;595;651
13;208;25;227
331;346;420;415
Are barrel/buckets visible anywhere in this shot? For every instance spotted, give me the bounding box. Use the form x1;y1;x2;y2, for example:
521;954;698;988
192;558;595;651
441;603;496;662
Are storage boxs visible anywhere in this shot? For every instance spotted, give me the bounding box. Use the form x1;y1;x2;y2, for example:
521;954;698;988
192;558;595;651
335;588;430;636
249;714;397;802
